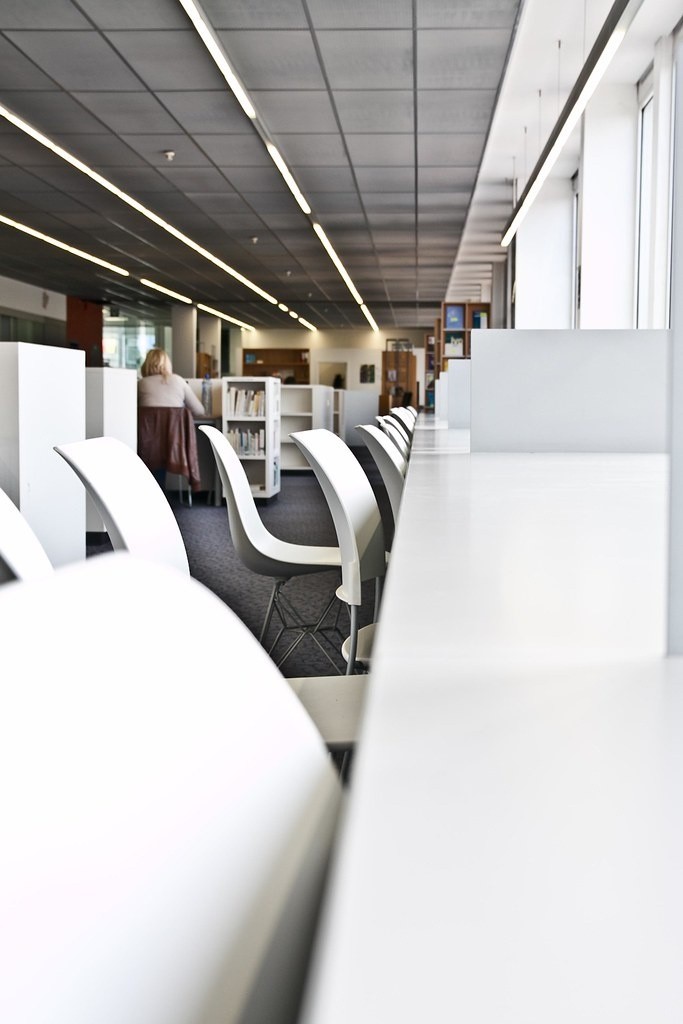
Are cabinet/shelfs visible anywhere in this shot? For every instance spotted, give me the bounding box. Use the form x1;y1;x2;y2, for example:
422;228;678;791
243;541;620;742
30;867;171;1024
424;301;491;411
0;340;346;572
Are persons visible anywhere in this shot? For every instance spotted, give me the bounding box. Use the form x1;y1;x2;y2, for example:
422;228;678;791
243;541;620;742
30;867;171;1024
138;348;205;416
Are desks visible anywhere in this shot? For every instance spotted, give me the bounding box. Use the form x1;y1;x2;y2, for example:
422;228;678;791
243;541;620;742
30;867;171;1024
294;410;682;1024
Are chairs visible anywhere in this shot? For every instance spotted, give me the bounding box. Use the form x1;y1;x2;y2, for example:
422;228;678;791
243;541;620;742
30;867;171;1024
0;406;417;1024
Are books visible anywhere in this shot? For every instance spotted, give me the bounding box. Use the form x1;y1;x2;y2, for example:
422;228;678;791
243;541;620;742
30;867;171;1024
227;428;264;456
227;387;265;417
